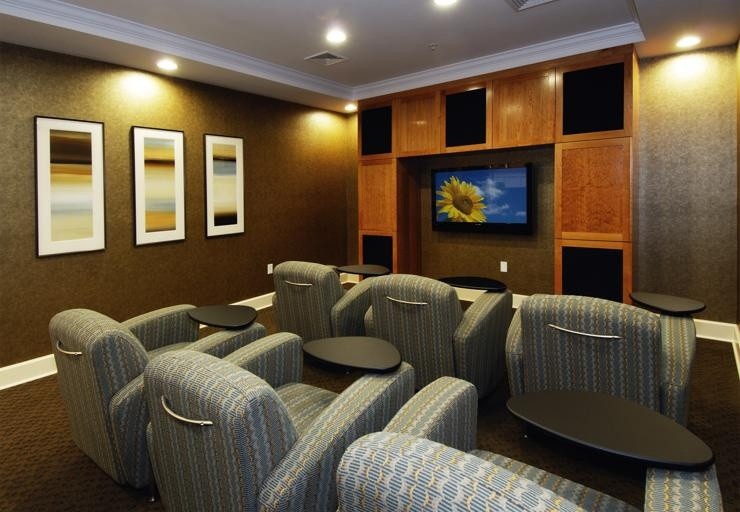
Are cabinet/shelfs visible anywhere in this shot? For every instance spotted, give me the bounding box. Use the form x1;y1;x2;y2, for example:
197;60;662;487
357;43;639;303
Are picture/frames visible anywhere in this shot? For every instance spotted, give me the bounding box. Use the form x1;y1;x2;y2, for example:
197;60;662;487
203;132;245;240
34;115;107;260
131;125;187;248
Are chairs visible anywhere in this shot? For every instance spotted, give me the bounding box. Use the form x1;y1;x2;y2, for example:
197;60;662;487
145;332;415;512
365;272;511;402
271;257;388;340
49;303;267;490
334;375;724;512
506;289;706;425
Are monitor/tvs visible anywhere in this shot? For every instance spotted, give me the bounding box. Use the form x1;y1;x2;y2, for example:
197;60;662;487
430;163;538;236
